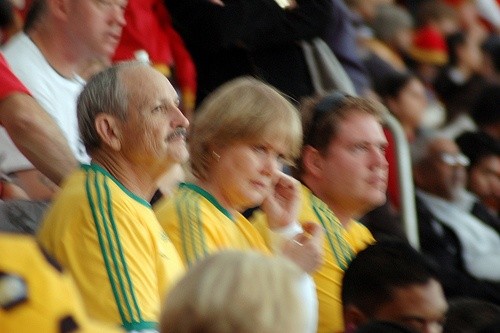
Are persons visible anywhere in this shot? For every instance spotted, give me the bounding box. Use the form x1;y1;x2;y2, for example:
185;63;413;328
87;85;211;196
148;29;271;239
1;0;132;203
114;0;500;333
252;91;390;333
0;52;80;203
341;237;448;333
152;75;323;278
36;60;189;333
158;249;320;333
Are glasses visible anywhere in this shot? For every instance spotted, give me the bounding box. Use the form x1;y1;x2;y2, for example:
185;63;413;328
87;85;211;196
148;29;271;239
427;153;470;167
